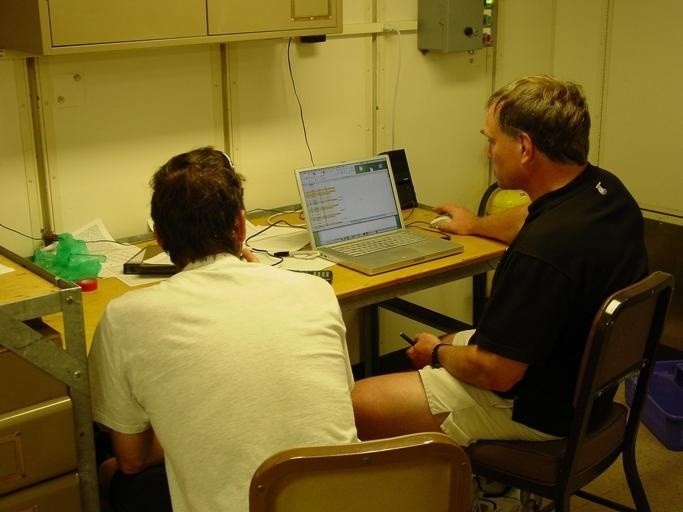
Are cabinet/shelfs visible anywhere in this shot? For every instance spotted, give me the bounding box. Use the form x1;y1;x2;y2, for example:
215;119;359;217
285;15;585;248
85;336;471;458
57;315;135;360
0;0;343;57
1;244;100;509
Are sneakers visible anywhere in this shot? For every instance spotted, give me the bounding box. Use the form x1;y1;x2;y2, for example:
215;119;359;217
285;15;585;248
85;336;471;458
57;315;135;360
472;477;543;512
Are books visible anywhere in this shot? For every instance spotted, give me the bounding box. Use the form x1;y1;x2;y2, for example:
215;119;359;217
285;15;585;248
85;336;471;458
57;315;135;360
122;246;180;274
141;243;177;269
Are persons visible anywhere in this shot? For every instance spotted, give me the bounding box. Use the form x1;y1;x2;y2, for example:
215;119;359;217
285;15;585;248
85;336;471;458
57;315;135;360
350;73;651;446
88;145;362;511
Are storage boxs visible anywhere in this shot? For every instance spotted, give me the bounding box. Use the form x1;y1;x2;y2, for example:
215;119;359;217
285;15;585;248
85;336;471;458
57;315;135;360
626;359;683;450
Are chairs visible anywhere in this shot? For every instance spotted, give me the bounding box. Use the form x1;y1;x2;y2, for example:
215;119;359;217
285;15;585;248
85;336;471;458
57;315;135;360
249;431;473;512
468;271;671;510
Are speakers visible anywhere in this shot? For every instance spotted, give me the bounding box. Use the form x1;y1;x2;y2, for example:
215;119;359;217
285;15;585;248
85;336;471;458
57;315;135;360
380;148;419;210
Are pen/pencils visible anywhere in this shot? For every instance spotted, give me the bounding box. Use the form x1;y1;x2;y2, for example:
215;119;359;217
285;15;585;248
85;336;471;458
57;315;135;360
400;332;415;346
274;251;320;257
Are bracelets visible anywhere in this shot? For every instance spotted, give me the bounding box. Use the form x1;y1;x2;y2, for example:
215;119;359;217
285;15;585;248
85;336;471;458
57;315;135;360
430;342;455;369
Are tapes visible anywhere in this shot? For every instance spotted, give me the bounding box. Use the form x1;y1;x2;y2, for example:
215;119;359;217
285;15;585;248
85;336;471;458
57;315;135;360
77;278;97;292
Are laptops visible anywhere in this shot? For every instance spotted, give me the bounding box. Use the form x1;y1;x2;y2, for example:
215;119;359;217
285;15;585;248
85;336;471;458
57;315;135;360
295;154;463;276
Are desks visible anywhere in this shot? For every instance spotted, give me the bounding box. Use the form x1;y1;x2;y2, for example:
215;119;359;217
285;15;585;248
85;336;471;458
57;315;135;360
35;203;512;511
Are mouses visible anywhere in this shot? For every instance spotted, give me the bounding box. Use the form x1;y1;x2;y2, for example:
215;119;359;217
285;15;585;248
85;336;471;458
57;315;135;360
428;214;451;229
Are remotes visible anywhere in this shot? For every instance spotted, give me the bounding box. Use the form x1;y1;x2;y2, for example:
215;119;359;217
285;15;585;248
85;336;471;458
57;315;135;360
290;269;333;283
